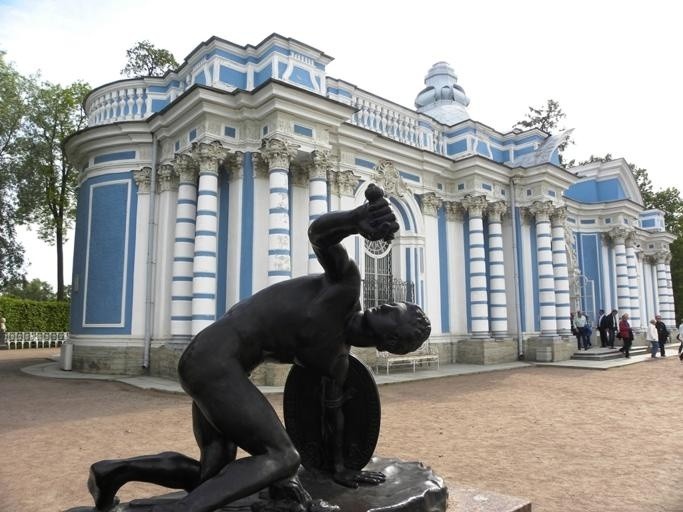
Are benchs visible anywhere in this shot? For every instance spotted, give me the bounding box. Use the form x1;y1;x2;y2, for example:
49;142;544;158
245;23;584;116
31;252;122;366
376;340;440;375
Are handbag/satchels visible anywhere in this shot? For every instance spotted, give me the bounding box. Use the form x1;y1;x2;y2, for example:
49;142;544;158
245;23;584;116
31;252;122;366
616;332;624;341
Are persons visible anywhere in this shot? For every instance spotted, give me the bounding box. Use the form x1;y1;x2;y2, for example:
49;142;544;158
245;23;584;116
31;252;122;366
646;319;660;358
83;194;432;511
569;310;592;351
597;309;609;347
655;315;670;357
606;309;618;349
618;312;634;358
676;318;683;361
